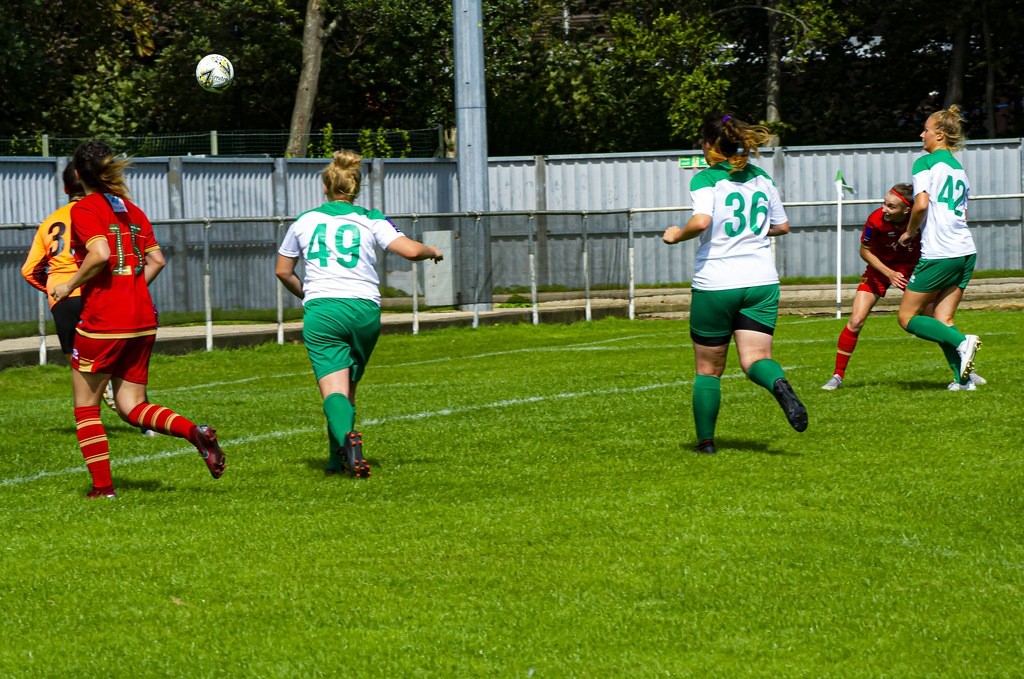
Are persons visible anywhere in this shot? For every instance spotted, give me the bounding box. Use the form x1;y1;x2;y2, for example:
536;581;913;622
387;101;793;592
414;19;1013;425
661;110;811;454
19;158;90;362
273;148;444;480
819;183;985;390
51;139;228;499
897;105;982;390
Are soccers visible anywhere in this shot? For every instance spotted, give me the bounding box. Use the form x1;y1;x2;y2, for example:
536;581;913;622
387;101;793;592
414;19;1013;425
196;53;235;94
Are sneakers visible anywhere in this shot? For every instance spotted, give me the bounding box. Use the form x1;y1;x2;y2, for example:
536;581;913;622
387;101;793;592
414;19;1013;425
957;334;982;379
694;437;716;454
964;369;987;386
774;378;807;433
822;373;843;389
84;488;117;498
342;425;371;481
195;422;225;478
947;381;976;392
102;377;121;410
